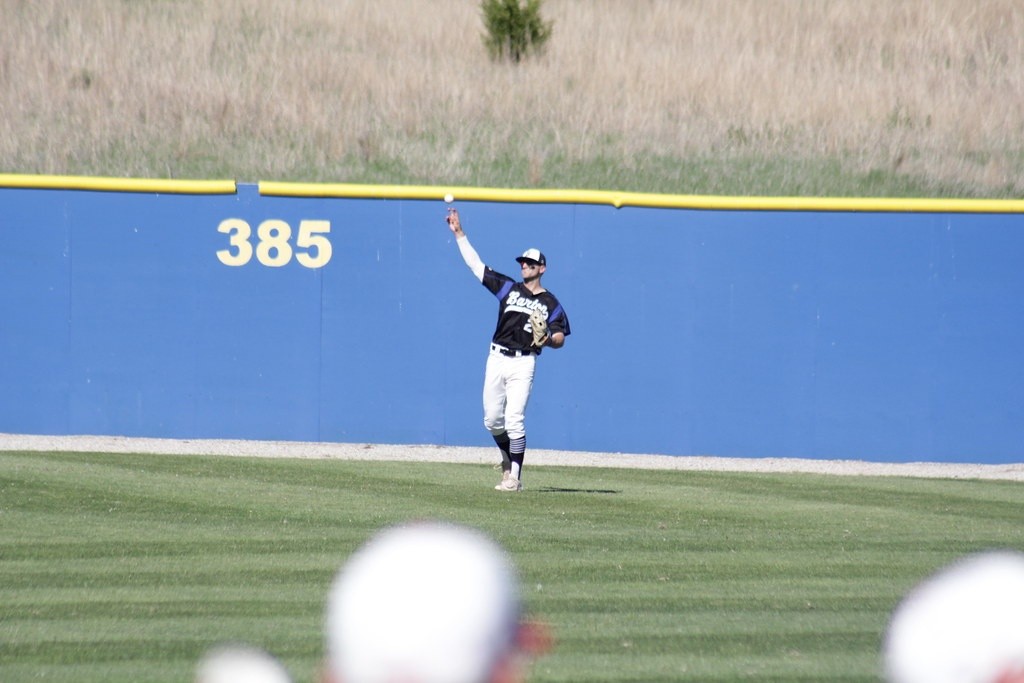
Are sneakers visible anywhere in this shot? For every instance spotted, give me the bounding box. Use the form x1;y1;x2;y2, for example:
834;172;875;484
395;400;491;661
503;475;523;491
495;473;509;490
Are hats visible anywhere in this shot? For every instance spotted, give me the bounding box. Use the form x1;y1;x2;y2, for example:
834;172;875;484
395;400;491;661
884;551;1024;683
516;248;546;266
322;522;553;683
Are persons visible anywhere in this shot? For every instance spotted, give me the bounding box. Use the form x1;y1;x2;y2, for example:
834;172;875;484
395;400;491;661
190;520;556;682
882;547;1024;683
445;208;573;492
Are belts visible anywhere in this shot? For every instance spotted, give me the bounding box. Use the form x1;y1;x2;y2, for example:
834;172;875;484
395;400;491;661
492;345;531;357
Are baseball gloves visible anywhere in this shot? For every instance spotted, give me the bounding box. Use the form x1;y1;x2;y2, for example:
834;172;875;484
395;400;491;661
529;309;552;348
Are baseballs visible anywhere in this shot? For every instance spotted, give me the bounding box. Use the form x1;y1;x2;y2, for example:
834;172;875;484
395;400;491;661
443;193;455;203
